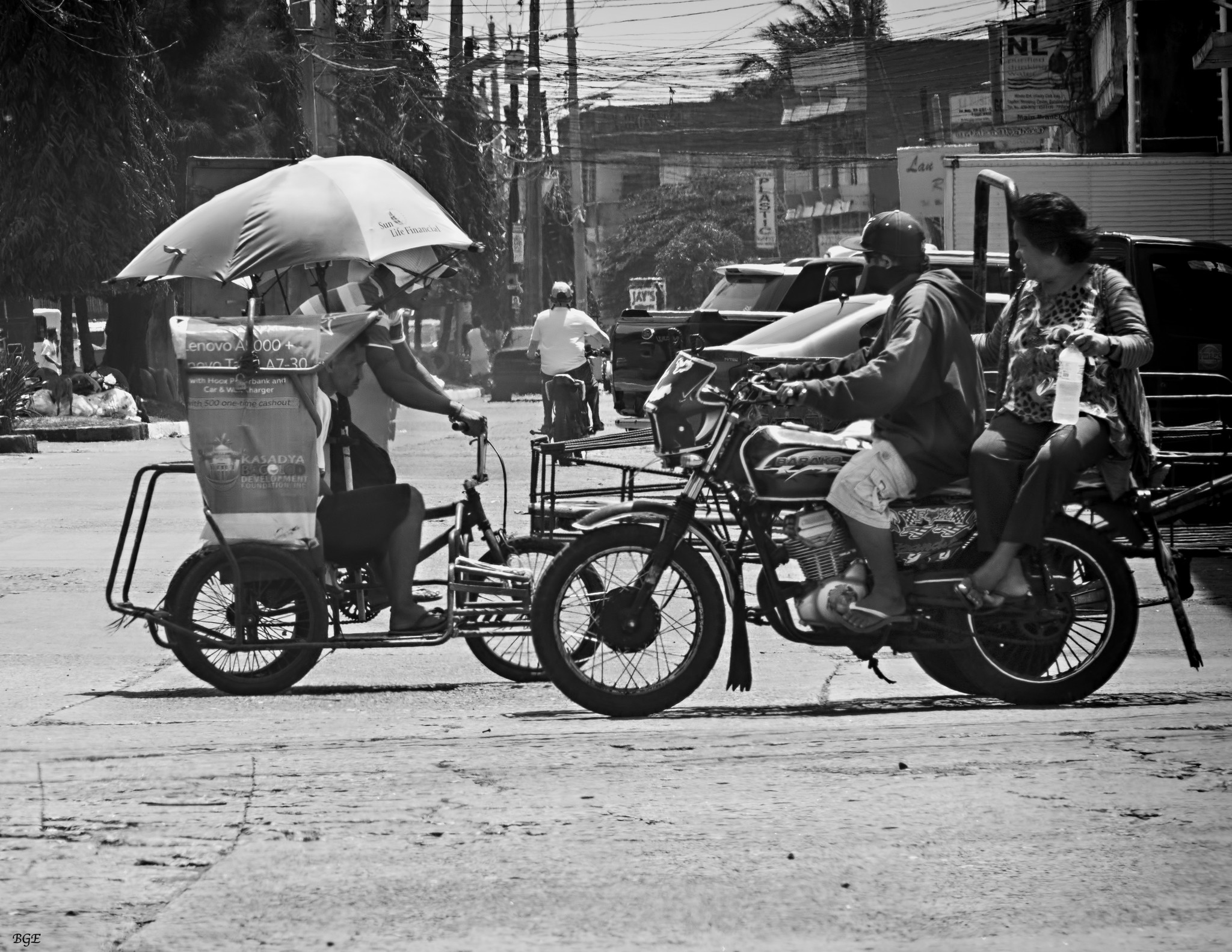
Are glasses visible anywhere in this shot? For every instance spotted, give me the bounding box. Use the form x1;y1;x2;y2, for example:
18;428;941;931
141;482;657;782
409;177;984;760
387;263;433;288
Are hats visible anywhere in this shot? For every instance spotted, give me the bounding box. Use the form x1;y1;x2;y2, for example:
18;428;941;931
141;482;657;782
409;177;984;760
374;244;459;279
839;210;926;257
551;282;573;302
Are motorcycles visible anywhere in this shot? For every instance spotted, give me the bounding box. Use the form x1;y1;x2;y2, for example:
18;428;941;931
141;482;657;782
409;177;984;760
523;338;1141;719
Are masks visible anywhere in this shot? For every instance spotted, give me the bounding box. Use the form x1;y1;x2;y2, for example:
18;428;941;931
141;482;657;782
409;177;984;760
866;265;895;295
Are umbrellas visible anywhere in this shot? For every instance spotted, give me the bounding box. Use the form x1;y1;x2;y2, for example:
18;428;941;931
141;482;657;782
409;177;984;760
100;147;481;314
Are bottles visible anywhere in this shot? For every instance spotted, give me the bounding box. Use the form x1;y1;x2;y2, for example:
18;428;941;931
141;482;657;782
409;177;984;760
1052;342;1086;425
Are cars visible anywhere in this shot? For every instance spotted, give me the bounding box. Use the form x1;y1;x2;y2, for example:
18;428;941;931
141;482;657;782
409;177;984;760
602;232;1232;521
488;325;545;400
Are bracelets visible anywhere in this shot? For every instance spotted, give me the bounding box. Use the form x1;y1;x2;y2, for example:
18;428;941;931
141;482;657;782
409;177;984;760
448;402;464;424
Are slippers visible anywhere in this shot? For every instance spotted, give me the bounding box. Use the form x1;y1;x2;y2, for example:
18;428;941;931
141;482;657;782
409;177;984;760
954;574;984;612
981;590;1031;611
370;587;443;612
389;607;448;636
839;602;911;632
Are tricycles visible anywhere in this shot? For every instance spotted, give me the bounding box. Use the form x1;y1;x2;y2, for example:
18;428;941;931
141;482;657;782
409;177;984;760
100;156;604;697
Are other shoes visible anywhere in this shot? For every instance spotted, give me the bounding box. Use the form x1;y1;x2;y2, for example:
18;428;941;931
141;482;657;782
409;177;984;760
594;421;605;431
541;424;552;433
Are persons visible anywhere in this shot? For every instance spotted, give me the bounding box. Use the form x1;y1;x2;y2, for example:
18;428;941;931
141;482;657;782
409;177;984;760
314;330;448;634
463;315;492;394
40;325;62;376
763;211;988;629
955;193;1153;610
525;281;610;433
323;247;486;615
